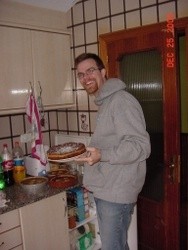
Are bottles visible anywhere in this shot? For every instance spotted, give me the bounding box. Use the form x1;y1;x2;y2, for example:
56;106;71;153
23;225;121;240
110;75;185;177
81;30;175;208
0;155;5;189
12;142;25;183
1;144;14;186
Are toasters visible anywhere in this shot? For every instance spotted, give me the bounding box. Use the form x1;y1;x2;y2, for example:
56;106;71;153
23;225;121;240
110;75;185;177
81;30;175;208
24;155;50;177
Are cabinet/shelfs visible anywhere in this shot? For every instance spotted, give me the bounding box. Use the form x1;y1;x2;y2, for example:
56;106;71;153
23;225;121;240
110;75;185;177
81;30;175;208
20;185;103;250
0;22;74;115
0;209;23;250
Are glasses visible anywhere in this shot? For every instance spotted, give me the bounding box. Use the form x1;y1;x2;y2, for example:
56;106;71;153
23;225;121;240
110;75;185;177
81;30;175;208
76;68;99;79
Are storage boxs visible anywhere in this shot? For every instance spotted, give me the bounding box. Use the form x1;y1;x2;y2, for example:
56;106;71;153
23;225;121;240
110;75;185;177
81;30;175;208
67;186;94;250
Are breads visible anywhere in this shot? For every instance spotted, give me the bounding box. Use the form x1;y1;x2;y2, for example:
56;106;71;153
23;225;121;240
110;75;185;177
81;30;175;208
47;142;86;159
21;178;46;185
49;169;67;175
51;176;76;182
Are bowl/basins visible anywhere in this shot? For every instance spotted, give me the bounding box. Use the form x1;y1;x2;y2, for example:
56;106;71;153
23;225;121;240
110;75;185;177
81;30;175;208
48;175;77;189
19;176;48;190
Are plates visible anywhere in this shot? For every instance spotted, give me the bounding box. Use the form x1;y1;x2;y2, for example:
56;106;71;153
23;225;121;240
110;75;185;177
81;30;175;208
47;151;88;163
46;169;68;178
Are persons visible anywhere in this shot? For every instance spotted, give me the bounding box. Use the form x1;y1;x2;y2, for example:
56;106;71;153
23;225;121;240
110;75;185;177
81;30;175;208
73;52;151;250
